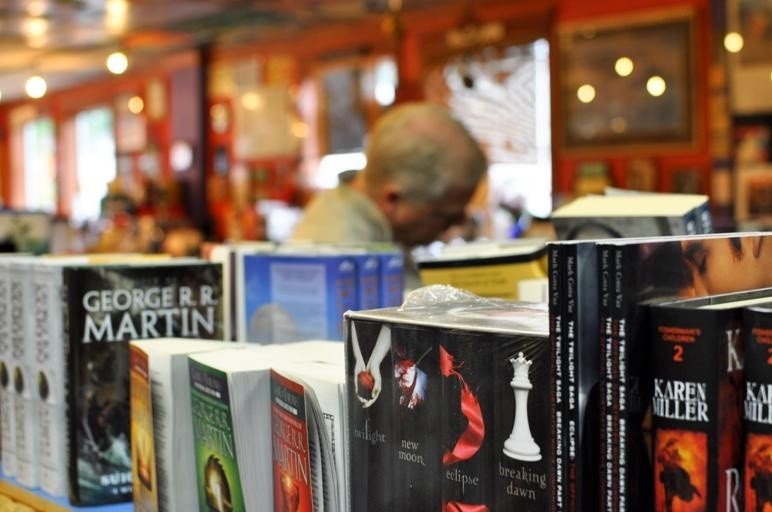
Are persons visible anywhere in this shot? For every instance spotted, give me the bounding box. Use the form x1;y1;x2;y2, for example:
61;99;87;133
289;101;487;248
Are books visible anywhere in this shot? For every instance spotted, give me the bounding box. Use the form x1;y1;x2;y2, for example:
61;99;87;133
0;158;772;512
